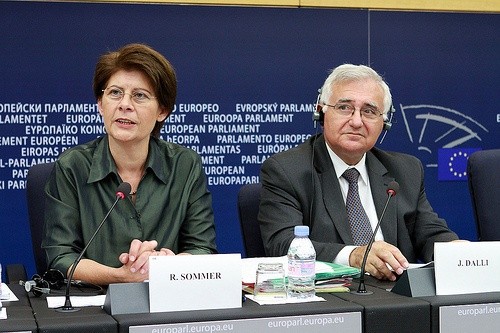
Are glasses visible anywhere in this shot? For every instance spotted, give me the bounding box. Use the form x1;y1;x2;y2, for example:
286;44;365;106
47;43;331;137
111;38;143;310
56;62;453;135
102;86;156;107
323;100;385;122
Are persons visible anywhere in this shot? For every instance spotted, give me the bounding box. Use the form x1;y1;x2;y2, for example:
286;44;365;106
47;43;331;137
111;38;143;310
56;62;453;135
40;44;218;286
256;62;471;281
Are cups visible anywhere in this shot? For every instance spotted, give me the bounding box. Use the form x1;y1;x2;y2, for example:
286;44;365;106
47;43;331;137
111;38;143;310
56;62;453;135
254;264;288;305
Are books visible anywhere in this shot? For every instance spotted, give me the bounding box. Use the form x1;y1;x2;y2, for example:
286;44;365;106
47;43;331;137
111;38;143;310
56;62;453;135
240;255;361;295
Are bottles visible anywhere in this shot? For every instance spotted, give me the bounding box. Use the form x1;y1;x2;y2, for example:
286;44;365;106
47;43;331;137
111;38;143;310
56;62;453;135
287;225;316;298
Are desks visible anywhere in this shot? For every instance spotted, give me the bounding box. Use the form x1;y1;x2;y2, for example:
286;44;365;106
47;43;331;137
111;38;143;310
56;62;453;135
0;263;500;333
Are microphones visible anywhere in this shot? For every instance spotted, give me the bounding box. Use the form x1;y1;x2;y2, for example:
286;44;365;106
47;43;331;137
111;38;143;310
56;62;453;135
56;182;131;312
350;181;400;295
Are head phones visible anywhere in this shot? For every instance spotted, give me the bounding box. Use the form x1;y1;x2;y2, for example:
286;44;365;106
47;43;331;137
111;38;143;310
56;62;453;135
313;86;395;133
25;268;103;296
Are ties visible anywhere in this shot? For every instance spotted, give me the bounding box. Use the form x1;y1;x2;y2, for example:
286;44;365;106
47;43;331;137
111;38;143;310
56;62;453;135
342;167;375;245
